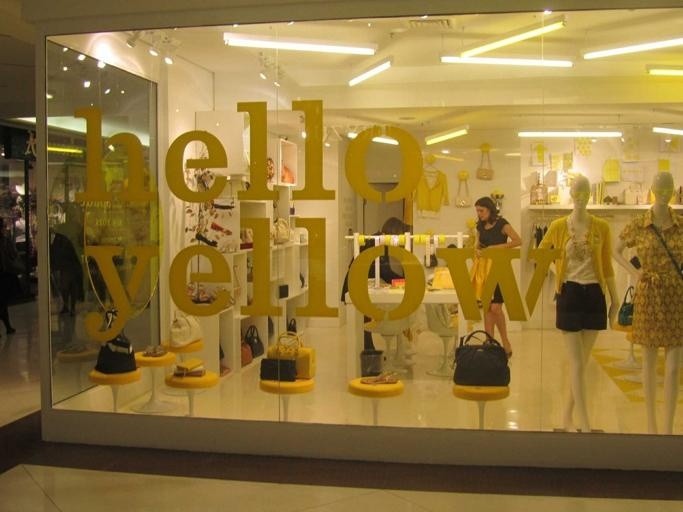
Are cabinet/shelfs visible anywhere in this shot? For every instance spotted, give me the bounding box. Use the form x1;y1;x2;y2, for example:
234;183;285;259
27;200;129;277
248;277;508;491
185;108;310;384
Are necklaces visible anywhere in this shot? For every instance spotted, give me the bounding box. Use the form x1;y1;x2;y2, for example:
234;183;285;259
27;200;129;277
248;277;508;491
567;215;591;260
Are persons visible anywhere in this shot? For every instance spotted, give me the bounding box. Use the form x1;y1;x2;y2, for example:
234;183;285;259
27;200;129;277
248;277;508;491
533;175;621;432
0;216;16;338
473;197;522;358
610;170;682;436
339;216;412;349
48;226;85;316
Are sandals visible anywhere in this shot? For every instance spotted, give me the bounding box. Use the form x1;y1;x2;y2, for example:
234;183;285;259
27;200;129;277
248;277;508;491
141;342;167;358
185;283;236;308
359;370;400;386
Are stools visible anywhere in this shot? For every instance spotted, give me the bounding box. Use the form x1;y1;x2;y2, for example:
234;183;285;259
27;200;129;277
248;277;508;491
88;365;139;415
164;373;220;418
160;342;208;396
128;349;180;415
451;382;510;429
348;376;406;426
378;316;418;378
258;376;314;423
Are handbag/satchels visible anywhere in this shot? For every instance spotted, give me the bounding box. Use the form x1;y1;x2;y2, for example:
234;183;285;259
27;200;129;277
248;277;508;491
281;160;295;184
617;284;636;326
172;356;207;379
166;306;205;353
93;307;137;375
240;226;255;249
286;317;298;332
239;323;264;368
268;201;290;245
452;328;512;386
451;167;683;209
257;330;318;383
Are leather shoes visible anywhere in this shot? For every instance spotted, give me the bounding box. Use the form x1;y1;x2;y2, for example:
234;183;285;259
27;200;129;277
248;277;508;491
210;222;233;236
194;231;218;247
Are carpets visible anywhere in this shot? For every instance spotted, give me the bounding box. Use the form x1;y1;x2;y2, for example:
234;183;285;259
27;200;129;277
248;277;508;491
592;353;683;405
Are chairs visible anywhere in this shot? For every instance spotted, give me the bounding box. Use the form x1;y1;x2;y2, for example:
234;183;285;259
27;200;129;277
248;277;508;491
424;302;457;378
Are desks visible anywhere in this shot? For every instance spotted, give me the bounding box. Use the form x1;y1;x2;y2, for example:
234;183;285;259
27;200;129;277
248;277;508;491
345;286;468;380
